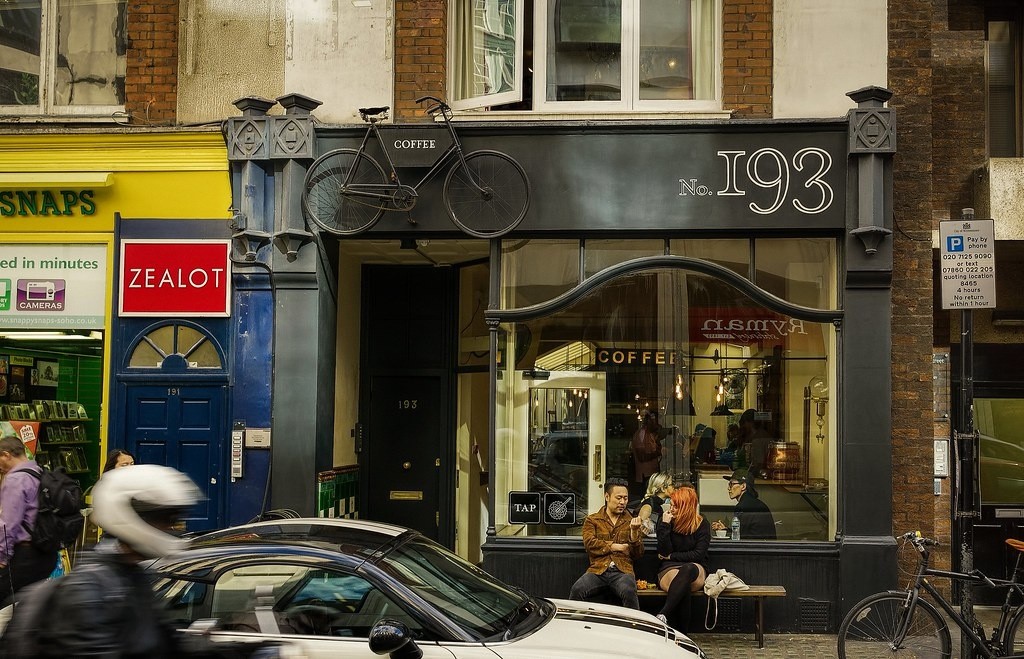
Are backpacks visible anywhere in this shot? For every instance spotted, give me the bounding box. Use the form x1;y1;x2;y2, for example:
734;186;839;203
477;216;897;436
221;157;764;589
6;463;85;555
0;564;131;659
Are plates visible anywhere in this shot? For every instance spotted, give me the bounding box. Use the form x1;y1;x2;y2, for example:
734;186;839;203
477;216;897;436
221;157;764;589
647;534;657;538
713;536;730;539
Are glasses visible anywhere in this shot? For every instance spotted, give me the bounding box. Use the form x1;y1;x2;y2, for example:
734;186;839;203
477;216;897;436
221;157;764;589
664;482;676;489
729;482;740;488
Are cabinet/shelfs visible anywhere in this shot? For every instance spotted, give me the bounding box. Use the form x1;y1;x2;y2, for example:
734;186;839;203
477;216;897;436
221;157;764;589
7;417;96;475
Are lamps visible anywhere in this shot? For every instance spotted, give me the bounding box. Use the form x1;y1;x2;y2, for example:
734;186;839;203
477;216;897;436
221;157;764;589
664;340;737;417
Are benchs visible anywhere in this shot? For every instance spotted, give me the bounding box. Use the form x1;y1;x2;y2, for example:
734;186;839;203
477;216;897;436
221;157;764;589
636;580;787;649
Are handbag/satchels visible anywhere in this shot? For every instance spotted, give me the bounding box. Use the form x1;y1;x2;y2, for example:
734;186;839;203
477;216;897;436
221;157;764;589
703;569;750;599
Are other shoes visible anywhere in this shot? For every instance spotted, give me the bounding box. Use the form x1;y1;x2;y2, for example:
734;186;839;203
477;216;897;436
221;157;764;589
656;614;667;625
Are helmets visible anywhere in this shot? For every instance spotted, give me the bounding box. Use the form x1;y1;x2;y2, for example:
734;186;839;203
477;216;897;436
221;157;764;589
89;464;204;559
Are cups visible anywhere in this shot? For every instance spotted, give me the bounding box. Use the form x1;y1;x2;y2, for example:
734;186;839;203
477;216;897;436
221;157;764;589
715;530;727;537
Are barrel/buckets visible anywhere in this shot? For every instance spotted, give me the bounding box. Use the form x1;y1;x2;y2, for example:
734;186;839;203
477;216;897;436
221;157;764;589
767;441;801;480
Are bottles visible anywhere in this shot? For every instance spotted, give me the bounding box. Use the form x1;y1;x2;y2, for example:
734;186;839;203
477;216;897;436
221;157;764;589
731;517;741;541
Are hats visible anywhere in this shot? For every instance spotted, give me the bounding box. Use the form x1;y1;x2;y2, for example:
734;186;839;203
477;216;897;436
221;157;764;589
722;469;755;488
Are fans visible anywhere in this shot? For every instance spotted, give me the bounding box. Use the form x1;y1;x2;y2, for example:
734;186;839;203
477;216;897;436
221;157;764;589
722;368;748;395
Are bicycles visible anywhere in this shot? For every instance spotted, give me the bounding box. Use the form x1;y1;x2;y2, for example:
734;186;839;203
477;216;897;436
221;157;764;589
302;94;532;238
836;528;1024;659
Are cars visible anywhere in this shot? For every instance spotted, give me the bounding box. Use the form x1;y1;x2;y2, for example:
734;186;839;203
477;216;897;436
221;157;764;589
522;461;588;526
1;518;709;659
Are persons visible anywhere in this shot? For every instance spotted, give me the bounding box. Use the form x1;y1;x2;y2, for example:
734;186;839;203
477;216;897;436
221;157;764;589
629;410;680;484
656;486;712;638
711;469;778;540
689;404;774;478
561;469;588;512
0;436;58;609
0;465;302;659
632;472;675;538
94;448;141;555
568;478;644;611
